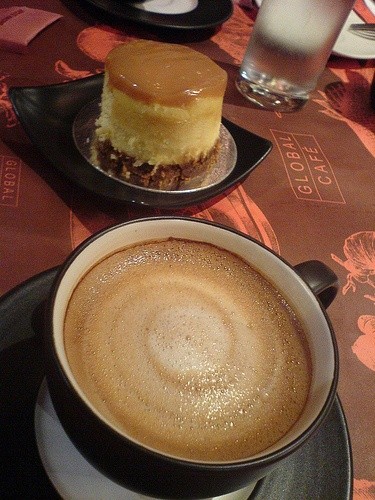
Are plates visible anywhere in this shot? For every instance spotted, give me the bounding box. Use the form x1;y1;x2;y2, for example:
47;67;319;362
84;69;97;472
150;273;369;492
0;265;352;500
255;0;375;59
88;0;234;31
7;70;274;209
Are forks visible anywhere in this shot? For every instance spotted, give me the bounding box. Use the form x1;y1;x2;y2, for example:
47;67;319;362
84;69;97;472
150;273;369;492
348;23;375;31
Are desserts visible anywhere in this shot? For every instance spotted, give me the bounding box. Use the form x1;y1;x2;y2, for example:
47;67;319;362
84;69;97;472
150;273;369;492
90;39;228;191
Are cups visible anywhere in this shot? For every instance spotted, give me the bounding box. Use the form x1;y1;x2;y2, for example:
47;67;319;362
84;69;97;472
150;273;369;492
234;0;356;113
46;214;340;500
